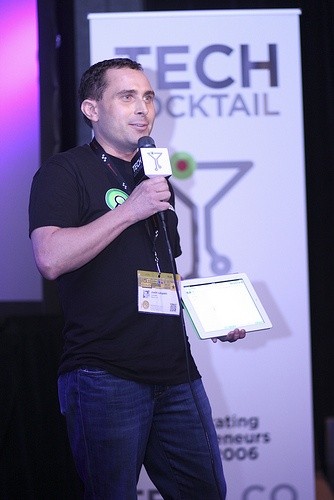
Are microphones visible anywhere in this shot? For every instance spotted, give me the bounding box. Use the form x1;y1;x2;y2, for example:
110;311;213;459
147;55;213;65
131;136;173;221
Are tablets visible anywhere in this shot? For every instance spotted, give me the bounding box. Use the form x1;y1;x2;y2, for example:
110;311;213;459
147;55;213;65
181;272;273;340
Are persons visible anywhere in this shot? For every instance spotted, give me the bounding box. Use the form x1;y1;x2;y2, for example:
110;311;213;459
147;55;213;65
28;58;247;500
